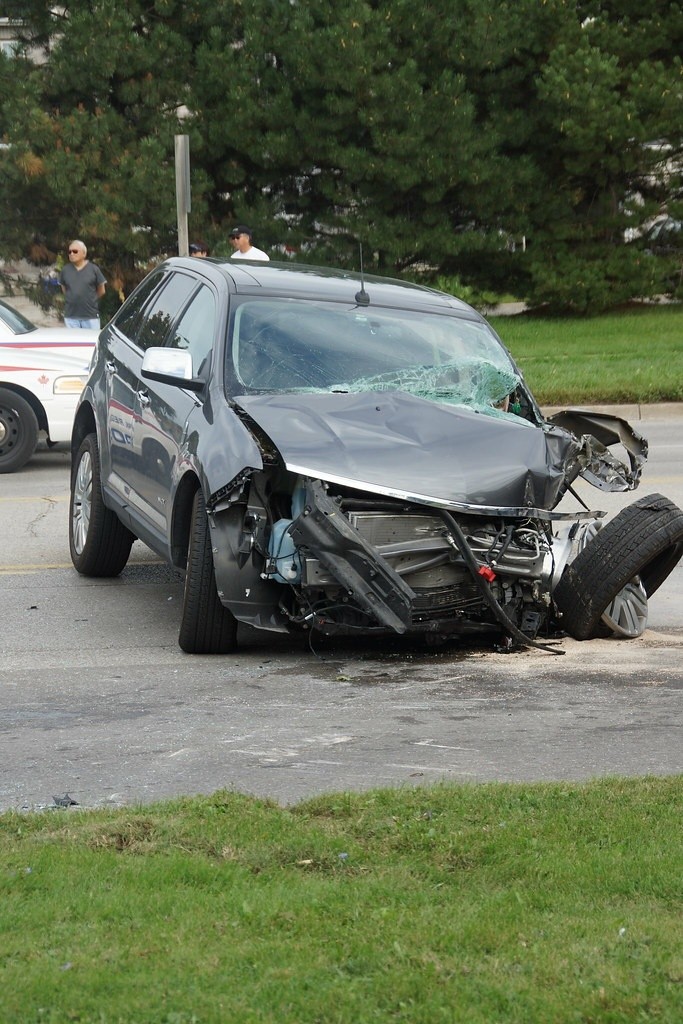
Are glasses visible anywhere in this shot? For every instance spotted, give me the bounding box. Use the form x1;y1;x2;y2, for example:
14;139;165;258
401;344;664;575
67;250;78;254
232;236;240;239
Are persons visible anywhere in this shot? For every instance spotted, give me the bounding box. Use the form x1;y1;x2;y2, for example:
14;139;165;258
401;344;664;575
190;241;210;257
56;239;107;330
228;225;270;262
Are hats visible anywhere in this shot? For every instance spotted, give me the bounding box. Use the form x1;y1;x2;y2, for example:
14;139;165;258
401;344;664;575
190;244;209;253
229;225;249;235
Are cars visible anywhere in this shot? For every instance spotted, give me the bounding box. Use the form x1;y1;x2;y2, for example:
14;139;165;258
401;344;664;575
68;257;683;658
0;299;103;474
